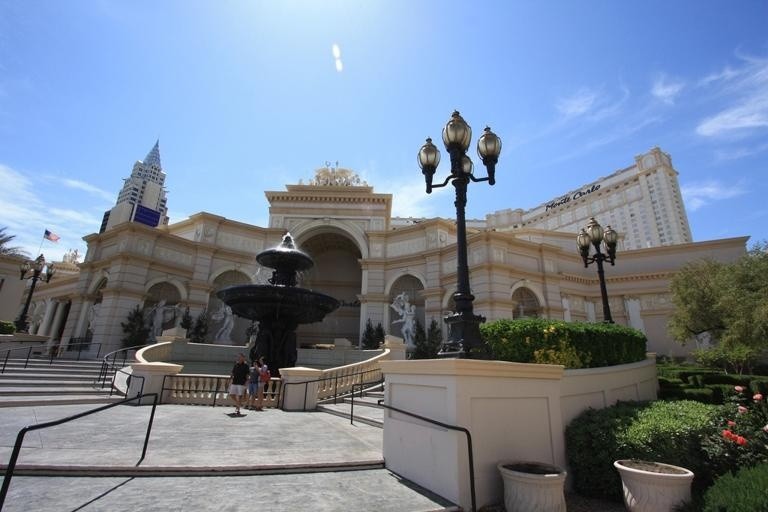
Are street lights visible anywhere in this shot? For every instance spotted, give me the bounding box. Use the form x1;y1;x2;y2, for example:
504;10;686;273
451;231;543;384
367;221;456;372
416;109;502;358
13;254;56;332
576;216;617;324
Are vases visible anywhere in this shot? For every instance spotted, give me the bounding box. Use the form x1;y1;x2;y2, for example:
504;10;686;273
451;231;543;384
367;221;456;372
614;460;694;512
497;458;567;512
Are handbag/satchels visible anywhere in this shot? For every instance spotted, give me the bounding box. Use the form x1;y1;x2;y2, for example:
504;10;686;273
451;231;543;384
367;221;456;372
260;370;271;382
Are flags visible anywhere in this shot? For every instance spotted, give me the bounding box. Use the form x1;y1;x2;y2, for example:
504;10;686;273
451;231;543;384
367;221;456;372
43;230;61;242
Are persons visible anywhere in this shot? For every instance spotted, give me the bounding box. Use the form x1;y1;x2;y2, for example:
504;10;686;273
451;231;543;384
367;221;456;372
401;304;416;342
144;297;177;338
243;359;260;410
254;356;269;411
215;306;236;339
226;352;250;417
389;290;417;326
